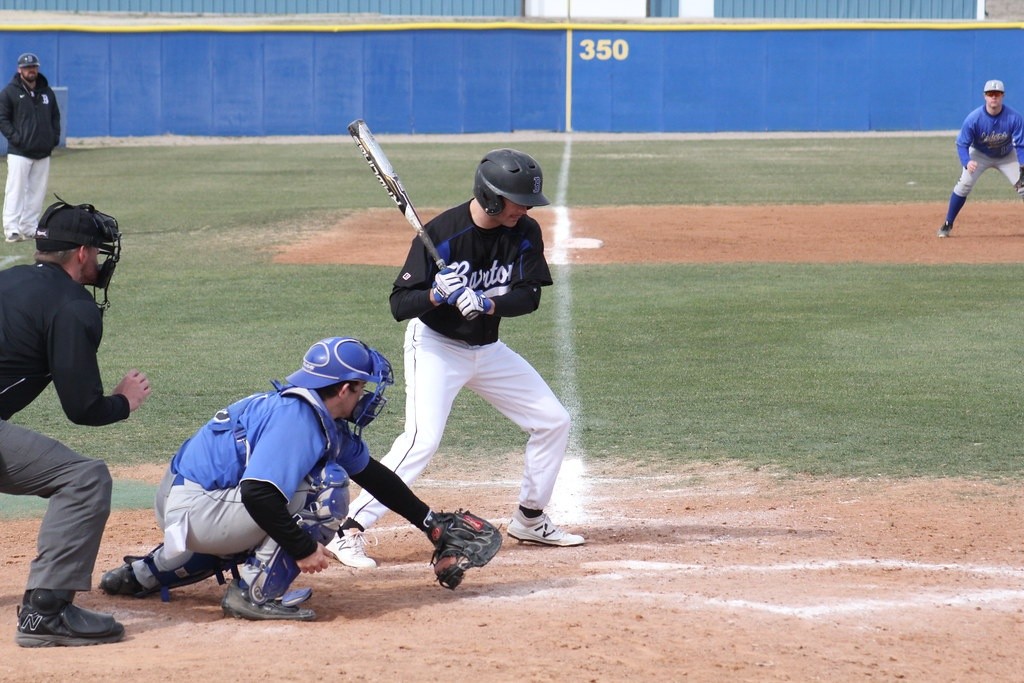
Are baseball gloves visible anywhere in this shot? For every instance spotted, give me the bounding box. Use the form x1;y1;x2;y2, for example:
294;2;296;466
425;507;503;592
1013;166;1024;200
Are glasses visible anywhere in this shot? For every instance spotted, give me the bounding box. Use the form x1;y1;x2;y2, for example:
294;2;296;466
985;91;1002;96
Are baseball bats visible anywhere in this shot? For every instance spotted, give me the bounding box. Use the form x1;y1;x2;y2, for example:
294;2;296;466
346;117;480;321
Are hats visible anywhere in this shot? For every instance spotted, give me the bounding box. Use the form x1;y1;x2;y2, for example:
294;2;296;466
34;191;118;252
984;80;1004;92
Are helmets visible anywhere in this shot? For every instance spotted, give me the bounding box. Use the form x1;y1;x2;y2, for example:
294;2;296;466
17;52;39;67
284;337;376;388
473;149;550;216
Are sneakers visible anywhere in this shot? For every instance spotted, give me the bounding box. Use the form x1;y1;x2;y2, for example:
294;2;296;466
325;530;377;568
221;579;317;620
99;562;154;596
15;603;125;648
507;509;585;546
5;232;22;243
936;224;950;238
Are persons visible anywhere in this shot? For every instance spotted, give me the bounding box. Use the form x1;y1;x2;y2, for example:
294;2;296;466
937;79;1024;238
323;148;585;569
0;52;61;243
99;337;502;622
0;193;150;648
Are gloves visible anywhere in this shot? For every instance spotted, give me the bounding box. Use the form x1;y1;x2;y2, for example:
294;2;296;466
431;260;470;304
1014;167;1024;193
447;285;491;316
